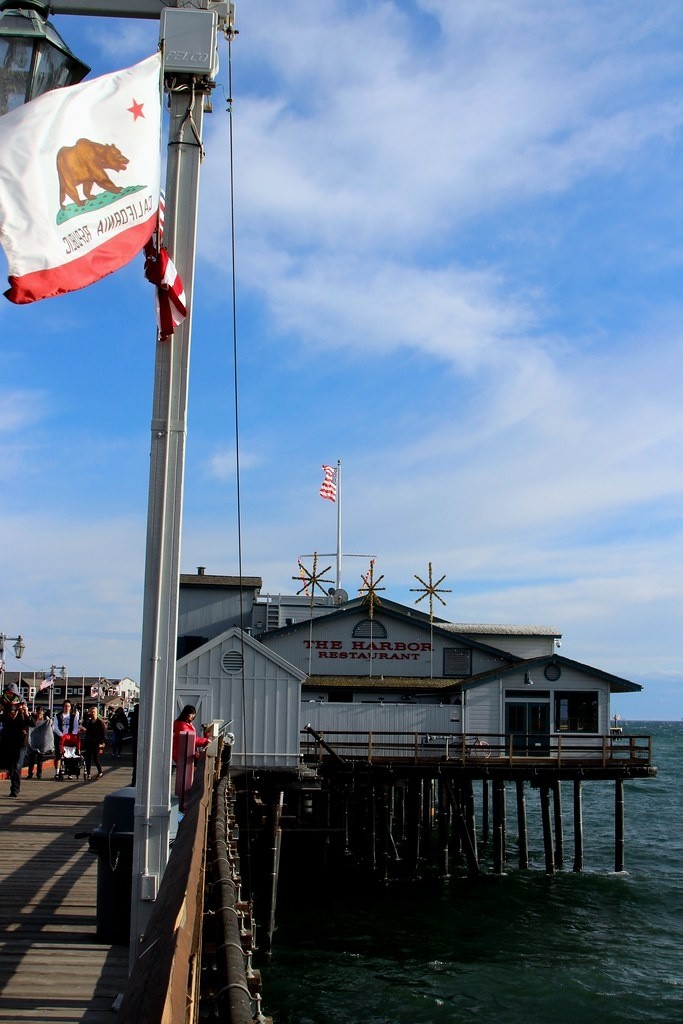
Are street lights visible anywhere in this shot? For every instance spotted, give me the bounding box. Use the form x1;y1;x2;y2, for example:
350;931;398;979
0;633;27;696
49;664;67;718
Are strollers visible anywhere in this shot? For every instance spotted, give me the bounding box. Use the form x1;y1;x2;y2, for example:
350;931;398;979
53;734;88;782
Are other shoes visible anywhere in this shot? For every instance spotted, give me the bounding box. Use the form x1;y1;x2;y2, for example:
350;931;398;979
9;790;17;797
68;776;73;779
95;773;103;780
37;775;41;779
127;784;135;787
27;775;33;779
87;774;92;780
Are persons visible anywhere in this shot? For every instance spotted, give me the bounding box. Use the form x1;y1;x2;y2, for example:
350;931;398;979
107;707;128;759
0;682;107;797
127;704;139;787
171;704;211;766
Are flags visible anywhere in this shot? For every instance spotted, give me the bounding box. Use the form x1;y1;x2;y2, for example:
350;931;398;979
320;464;337;502
1;53;187;339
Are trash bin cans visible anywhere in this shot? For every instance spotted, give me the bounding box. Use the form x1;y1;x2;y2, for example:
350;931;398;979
97;787;135;943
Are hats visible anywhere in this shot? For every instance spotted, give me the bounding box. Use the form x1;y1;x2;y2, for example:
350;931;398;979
3;683;19;697
